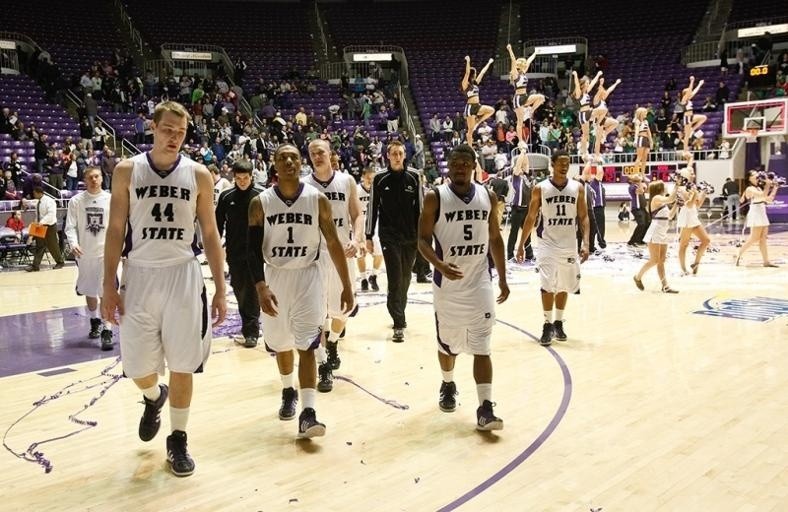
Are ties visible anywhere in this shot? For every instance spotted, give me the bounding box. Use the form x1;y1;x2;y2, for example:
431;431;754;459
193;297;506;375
38;201;41;224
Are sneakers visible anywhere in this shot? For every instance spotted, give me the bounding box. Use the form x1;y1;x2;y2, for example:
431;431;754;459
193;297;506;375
477;400;503;430
634;275;644;290
91;318;113;350
662;288;678;293
392;327;404;342
279;340;340;438
439;380;456;411
362;275;379;290
139;383;168;441
167;430;195;476
245;337;257;347
541;320;566;345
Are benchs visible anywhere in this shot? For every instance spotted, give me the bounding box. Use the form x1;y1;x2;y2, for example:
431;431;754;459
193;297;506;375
0;0;788;269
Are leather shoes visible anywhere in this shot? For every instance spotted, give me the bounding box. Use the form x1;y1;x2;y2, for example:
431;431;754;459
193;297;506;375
24;264;40;272
54;262;65;269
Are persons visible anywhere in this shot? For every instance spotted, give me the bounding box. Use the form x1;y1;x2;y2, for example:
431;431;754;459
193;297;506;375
0;30;787;352
98;101;224;476
248;143;354;438
298;139;365;395
416;145;510;433
